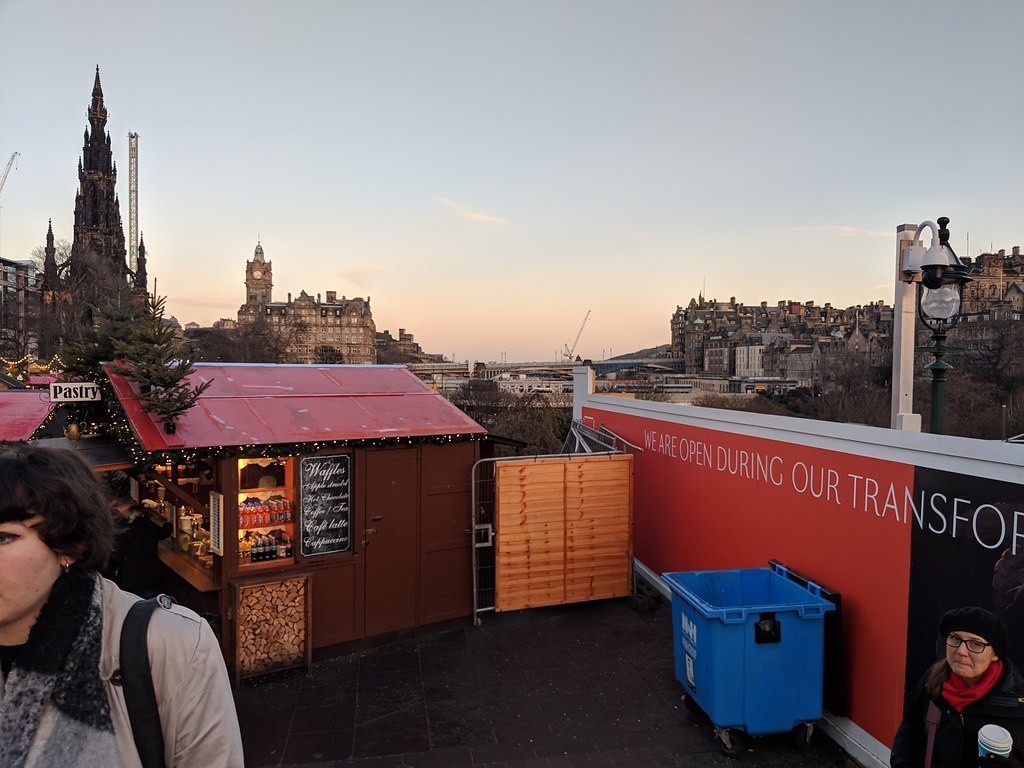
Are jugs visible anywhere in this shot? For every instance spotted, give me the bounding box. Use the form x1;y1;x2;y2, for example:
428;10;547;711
63;422;87;442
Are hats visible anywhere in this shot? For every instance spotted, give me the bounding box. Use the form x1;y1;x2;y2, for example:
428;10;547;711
939;606;1012;658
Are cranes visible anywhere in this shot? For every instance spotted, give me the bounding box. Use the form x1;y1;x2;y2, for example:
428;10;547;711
0;149;22;195
563;309;591;360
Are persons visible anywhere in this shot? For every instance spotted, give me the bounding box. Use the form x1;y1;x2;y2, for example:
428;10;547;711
0;443;246;768
107;518;174;600
890;607;1024;768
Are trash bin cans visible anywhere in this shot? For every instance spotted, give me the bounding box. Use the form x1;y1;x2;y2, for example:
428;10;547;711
661;561;842;755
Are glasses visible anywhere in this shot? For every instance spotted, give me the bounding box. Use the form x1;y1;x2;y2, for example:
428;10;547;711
945;632;992;653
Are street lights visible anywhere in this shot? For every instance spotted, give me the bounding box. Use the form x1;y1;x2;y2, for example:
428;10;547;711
916;217;974;434
890;221;960;434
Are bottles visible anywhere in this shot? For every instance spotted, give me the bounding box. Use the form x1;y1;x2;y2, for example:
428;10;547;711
192;518;199;531
159;497;166;513
237;500;294;565
